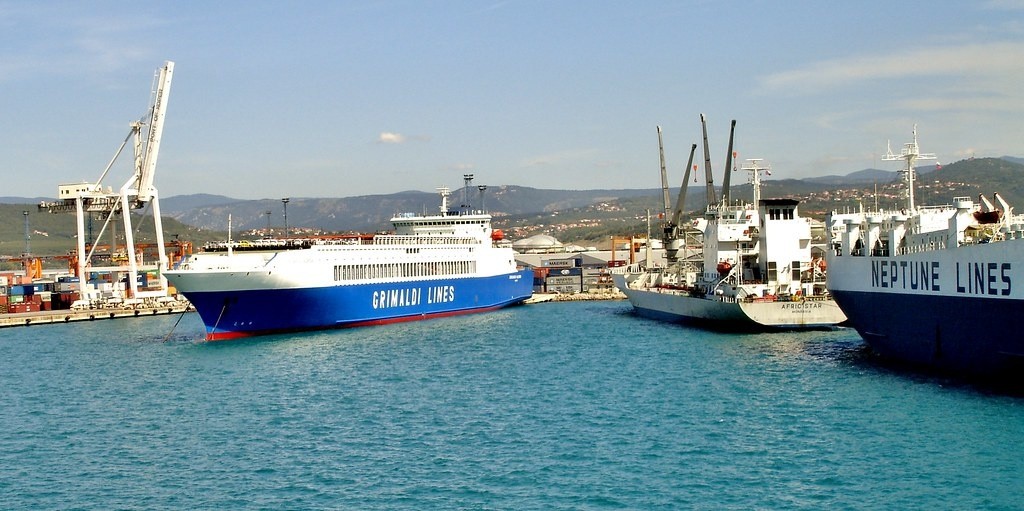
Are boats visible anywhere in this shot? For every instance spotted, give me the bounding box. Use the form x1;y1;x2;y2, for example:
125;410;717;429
606;194;849;330
823;124;1024;375
160;208;535;346
